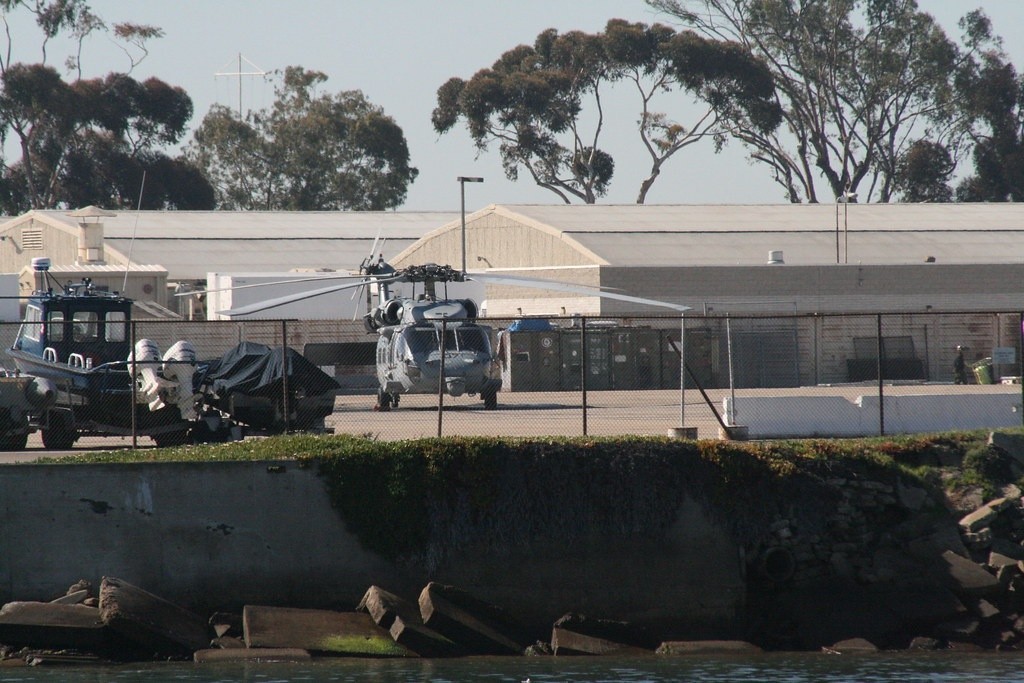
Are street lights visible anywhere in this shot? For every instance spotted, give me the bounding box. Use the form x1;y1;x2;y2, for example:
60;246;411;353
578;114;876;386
835;191;859;264
457;175;487;279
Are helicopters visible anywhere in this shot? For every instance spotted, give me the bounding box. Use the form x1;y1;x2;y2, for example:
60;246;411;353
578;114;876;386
165;267;694;414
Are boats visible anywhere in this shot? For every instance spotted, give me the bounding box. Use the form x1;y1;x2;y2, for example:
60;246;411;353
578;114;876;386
4;254;213;452
0;369;61;454
